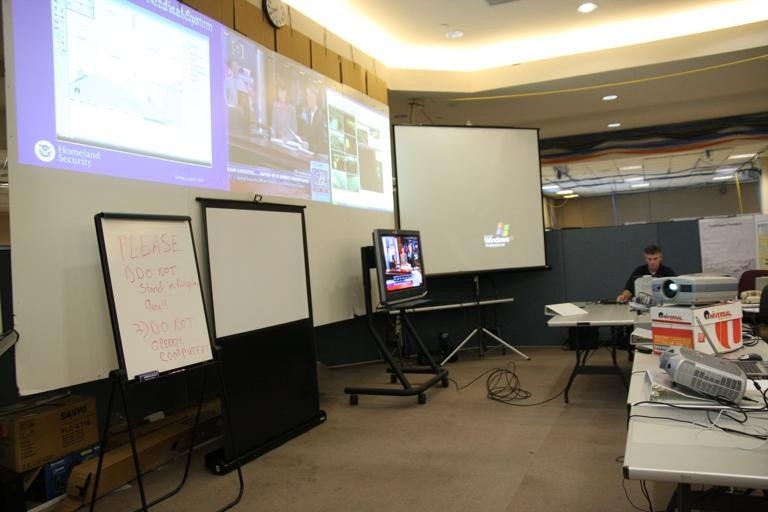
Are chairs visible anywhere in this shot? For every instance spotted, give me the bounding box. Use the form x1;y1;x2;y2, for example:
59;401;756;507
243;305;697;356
738;270;768;299
756;285;767;338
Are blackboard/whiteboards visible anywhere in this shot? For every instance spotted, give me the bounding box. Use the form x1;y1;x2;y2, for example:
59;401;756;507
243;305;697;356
97;213;216;385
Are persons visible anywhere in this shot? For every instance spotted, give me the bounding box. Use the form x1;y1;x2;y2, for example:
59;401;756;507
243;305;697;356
617;246;677;304
386;245;416;269
758;283;768;342
226;59;327;153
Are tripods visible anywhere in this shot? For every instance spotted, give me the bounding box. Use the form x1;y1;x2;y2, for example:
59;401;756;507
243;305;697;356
440;274;531;366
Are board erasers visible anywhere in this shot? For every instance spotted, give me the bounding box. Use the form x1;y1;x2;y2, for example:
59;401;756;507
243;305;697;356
135;370;159;381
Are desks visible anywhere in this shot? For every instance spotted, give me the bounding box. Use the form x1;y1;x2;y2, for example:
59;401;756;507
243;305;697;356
546;301;637;403
620;323;768;509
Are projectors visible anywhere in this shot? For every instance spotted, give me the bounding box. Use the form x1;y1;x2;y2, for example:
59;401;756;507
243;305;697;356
650;273;739;306
661;346;746;406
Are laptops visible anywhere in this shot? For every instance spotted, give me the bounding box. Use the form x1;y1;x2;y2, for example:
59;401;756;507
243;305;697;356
695;315;767;380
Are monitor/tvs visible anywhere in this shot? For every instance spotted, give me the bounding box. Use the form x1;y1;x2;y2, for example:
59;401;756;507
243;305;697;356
373;228;427;304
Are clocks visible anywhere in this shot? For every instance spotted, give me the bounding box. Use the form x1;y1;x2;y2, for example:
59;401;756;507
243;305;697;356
264;0;289;29
